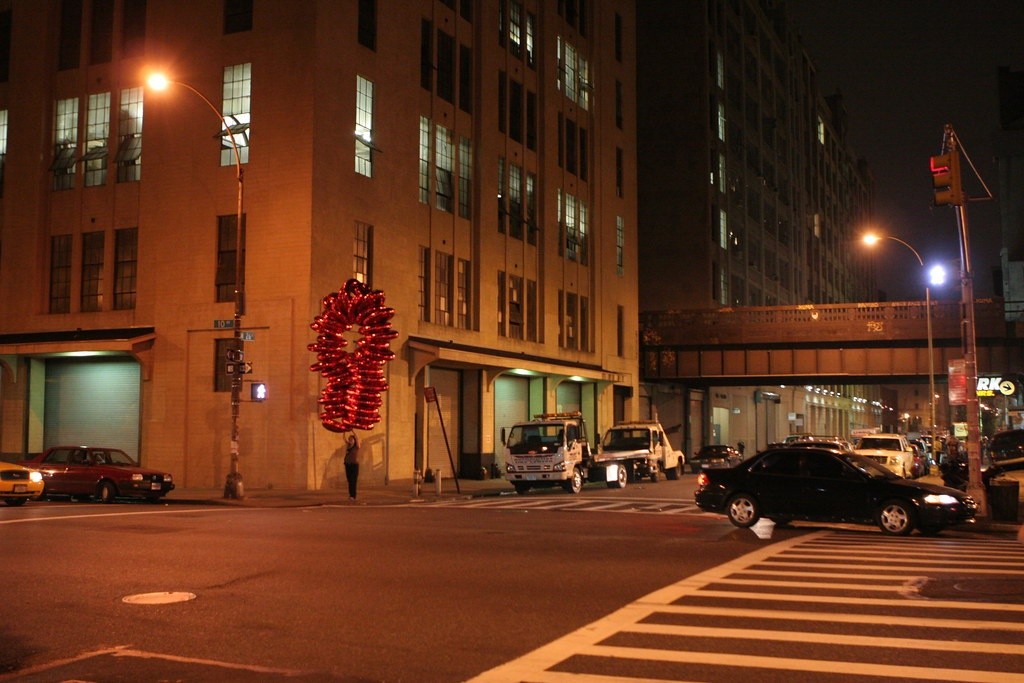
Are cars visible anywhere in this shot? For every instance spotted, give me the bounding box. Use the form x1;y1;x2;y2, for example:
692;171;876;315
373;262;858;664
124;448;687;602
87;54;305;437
981;428;1023;504
688;443;744;474
0;461;45;506
16;443;176;504
694;446;980;537
778;430;948;479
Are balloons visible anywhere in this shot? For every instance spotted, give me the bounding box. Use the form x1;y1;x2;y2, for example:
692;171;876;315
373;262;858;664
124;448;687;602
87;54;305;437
307;279;399;433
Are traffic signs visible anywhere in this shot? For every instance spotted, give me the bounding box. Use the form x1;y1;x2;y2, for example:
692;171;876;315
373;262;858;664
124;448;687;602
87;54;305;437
225;348;245;363
226;361;253;374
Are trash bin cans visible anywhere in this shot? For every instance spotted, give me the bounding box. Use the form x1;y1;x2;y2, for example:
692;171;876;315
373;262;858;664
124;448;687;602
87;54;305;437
989;478;1019;521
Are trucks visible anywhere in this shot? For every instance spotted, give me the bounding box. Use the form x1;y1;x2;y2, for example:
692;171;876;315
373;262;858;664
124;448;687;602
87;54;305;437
500;411;649;495
592;412;686;489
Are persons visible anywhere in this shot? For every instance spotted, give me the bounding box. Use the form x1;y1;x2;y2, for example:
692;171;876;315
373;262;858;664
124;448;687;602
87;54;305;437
343;430;361;500
737;440;746;455
935;431;961;456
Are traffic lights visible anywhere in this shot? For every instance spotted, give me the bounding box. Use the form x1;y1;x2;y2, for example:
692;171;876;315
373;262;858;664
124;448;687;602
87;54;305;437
249;381;268;401
930;151;963;206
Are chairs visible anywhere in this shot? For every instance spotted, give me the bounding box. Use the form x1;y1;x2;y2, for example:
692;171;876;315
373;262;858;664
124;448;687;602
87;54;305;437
527;435;541;446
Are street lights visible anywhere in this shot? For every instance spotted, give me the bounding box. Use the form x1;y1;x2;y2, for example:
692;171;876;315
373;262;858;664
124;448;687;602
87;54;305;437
144;71;248;499
860;231;938;464
